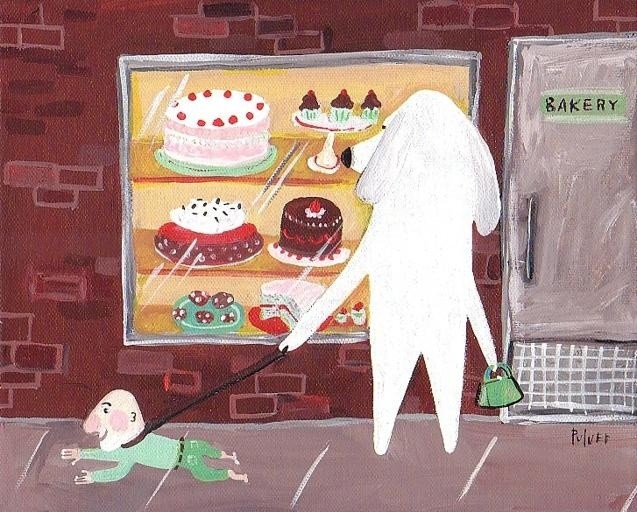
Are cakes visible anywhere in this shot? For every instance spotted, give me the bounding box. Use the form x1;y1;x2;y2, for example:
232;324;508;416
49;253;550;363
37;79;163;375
297;89;322;123
329;88;354;125
153;89;278;177
267;197;351;267
359;88;380;123
153;194;264;270
259;278;327;331
